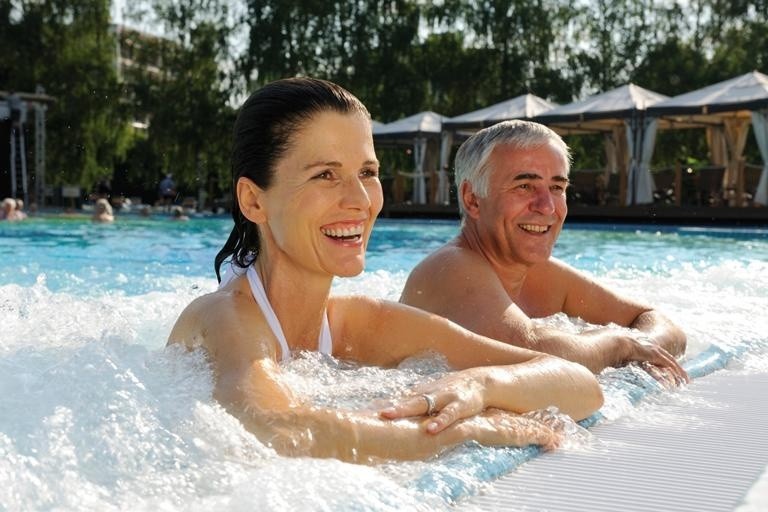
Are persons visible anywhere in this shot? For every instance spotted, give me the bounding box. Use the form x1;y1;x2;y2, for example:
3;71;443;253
400;118;689;387
0;167;230;221
161;76;604;463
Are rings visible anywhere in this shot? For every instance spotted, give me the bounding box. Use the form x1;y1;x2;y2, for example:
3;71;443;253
422;392;436;415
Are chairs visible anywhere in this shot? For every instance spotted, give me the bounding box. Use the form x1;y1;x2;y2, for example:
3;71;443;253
380;168;458;208
655;164;729;209
568;169;619;204
